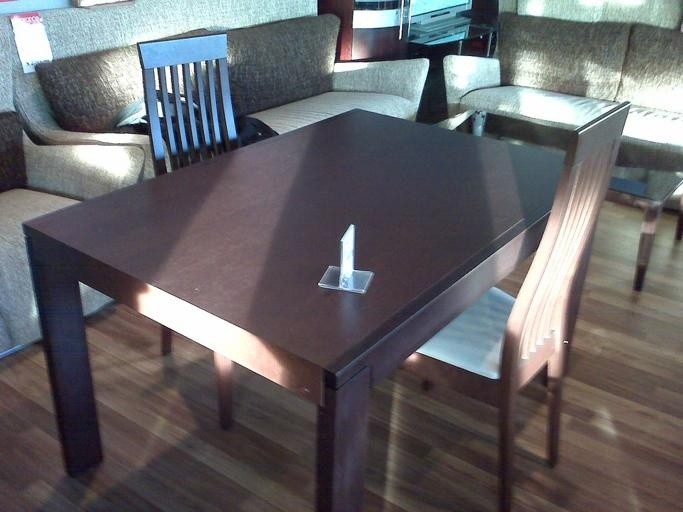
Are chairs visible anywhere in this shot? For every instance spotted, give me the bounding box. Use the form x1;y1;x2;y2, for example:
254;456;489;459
400;99;632;511
137;32;241;432
0;15;144;359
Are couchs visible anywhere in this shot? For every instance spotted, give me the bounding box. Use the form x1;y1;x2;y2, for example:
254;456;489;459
9;0;431;182
442;11;683;212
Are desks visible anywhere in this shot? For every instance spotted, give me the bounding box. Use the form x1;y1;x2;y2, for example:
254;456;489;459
22;107;598;512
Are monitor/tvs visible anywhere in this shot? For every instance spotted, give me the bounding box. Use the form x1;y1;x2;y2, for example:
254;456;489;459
408;0;473;33
410;18;472;54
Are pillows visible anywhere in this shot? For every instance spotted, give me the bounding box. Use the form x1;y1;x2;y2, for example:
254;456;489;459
214;13;341;118
35;28;229;133
0;112;27;191
